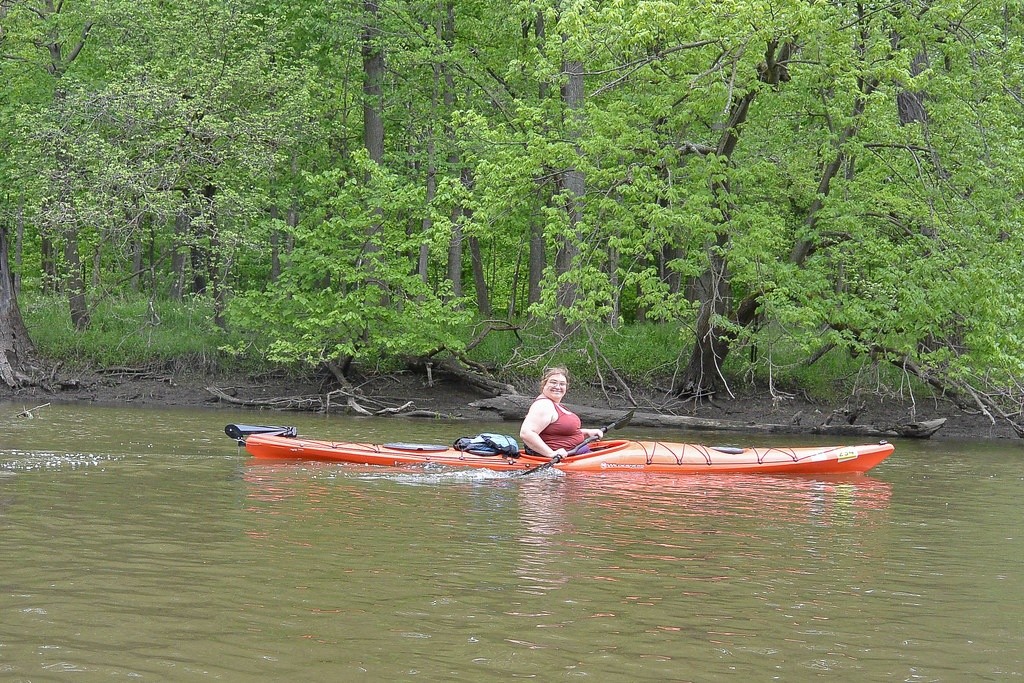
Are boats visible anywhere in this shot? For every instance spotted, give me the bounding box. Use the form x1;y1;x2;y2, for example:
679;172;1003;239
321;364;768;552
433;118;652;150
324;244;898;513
224;423;893;473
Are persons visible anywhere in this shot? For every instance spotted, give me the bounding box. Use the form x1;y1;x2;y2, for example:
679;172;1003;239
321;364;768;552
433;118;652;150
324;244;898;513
519;367;604;459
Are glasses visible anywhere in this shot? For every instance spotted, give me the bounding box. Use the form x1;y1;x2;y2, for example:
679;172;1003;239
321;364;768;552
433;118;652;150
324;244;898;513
546;380;569;389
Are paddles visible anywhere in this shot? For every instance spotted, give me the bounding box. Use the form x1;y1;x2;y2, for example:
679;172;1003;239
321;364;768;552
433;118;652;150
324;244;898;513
522;409;636;476
